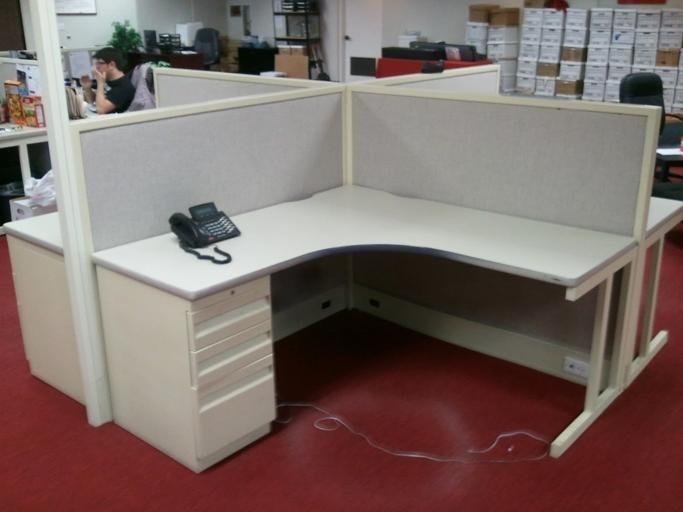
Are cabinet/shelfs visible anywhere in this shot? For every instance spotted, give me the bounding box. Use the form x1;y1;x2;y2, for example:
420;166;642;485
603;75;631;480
272;0;324;79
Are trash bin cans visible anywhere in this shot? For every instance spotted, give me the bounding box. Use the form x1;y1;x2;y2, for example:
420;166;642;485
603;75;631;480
0;181;25;227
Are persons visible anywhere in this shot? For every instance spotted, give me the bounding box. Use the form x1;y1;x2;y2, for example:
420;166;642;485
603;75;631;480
78;45;135;116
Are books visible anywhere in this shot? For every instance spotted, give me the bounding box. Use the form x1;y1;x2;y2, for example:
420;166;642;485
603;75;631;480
279;0;310;14
67;86;83;120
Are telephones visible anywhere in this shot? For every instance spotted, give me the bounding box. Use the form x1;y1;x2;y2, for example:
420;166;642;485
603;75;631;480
168;202;241;248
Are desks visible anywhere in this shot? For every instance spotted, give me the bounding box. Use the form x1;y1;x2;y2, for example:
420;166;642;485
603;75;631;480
0;46;683;474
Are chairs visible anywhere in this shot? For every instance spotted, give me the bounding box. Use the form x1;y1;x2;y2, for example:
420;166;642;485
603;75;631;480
194;29;221;71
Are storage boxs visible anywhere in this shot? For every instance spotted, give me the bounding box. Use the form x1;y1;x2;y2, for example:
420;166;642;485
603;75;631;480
466;1;683;117
